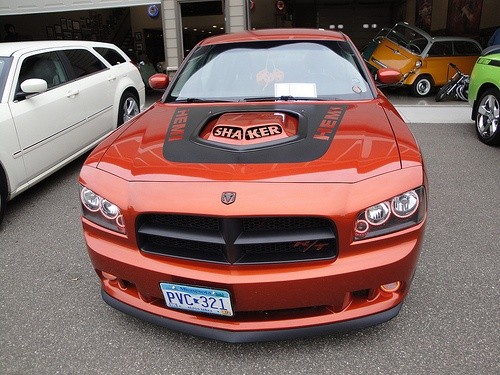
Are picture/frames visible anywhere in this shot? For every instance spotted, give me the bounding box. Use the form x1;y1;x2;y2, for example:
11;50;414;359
53;23;62;34
66;19;73;31
135;43;143;52
59;18;67;30
63;30;73;40
46;25;55;38
73;31;83;39
73;20;81;30
56;35;63;39
135;31;142;41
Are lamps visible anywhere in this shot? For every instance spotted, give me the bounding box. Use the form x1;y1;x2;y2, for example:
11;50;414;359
183;26;189;30
193;27;198;32
201;28;205;33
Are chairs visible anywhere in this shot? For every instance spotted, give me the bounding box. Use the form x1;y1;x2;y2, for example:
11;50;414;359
17;60;54;93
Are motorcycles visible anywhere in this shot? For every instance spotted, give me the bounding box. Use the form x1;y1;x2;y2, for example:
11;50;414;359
435;63;470;101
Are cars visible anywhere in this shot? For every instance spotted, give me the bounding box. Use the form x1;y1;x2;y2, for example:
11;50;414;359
358;21;500;145
77;29;429;343
0;40;145;222
